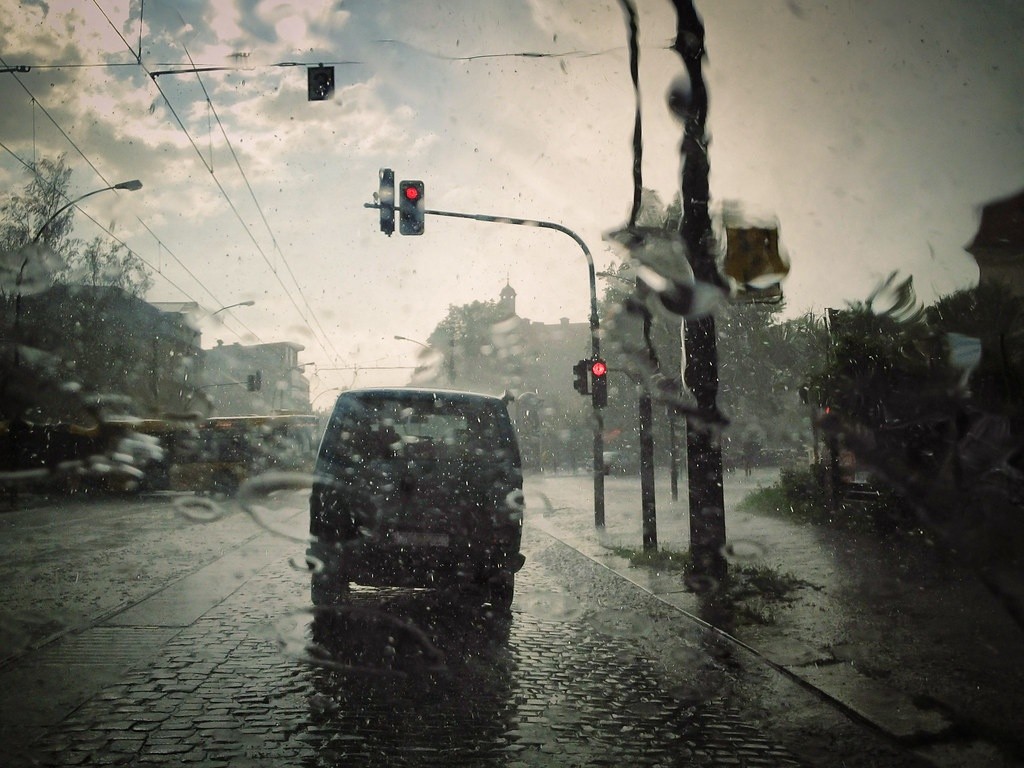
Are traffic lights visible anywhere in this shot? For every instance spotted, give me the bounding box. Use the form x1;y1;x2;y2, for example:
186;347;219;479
573;360;588;396
399;179;425;236
378;169;394;237
590;359;608;409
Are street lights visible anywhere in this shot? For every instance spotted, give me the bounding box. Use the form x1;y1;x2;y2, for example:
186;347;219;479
311;387;339;406
183;300;256;421
394;337;444;362
11;179;145;375
271;361;315;414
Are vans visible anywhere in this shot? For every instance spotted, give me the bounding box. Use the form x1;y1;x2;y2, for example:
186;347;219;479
304;387;527;631
44;413;320;501
